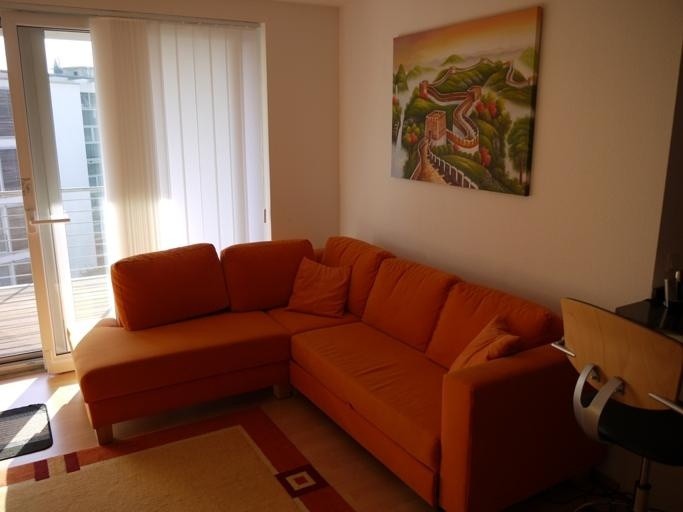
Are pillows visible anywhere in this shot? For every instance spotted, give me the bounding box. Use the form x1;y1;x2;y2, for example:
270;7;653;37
220;240;314;313
288;257;352;319
110;242;231;331
360;257;463;350
449;316;529;375
322;236;397;317
426;280;556;374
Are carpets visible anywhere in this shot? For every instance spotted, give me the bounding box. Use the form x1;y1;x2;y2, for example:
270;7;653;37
0;404;53;461
0;427;298;512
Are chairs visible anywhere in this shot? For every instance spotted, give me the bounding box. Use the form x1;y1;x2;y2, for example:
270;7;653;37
551;295;683;512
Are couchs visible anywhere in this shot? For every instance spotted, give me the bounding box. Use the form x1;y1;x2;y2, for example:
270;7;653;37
70;235;598;512
